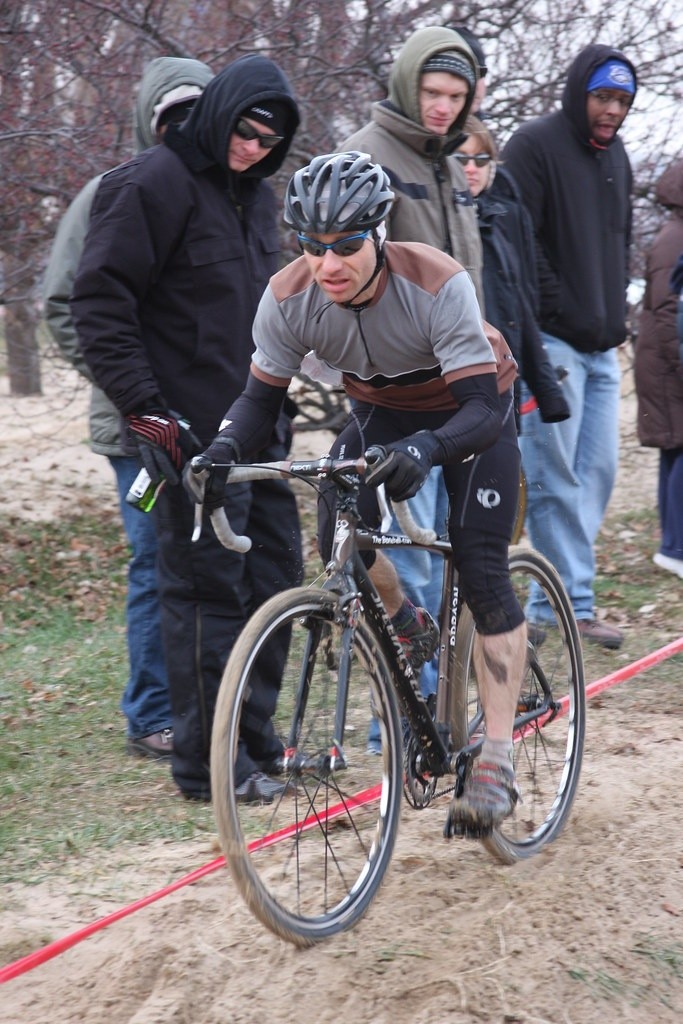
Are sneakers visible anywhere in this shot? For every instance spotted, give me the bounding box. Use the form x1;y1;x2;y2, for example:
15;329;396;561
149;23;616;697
449;753;518;828
371;607;440;721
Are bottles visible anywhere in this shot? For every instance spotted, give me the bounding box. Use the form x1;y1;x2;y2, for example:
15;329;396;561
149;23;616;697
125;419;191;512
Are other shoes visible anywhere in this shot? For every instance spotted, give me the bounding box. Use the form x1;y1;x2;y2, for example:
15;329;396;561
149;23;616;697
129;730;173;758
575;618;625;646
235;746;320;803
526;620;547;644
652;551;683;579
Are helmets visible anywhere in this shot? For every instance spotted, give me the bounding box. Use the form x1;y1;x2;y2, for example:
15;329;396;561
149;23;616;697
283;150;396;235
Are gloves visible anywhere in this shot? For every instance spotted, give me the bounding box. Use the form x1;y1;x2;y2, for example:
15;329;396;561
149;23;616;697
364;428;444;503
181;439;237;510
127;401;205;489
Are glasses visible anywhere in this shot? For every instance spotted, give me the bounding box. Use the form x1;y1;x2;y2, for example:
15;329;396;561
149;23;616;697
234;119;283;149
453;152;492;167
590;90;634;106
296;228;373;257
480;67;487;78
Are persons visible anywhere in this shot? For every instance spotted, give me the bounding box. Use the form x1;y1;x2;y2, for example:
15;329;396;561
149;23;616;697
636;148;683;579
319;20;573;780
179;151;529;843
41;53;304;809
486;42;637;653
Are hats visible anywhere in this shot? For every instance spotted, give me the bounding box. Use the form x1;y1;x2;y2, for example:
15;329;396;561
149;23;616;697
451;25;485;66
419;48;475;89
242;100;296;136
588;60;635;93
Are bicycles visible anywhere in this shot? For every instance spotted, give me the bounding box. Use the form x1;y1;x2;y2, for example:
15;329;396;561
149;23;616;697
190;455;586;949
